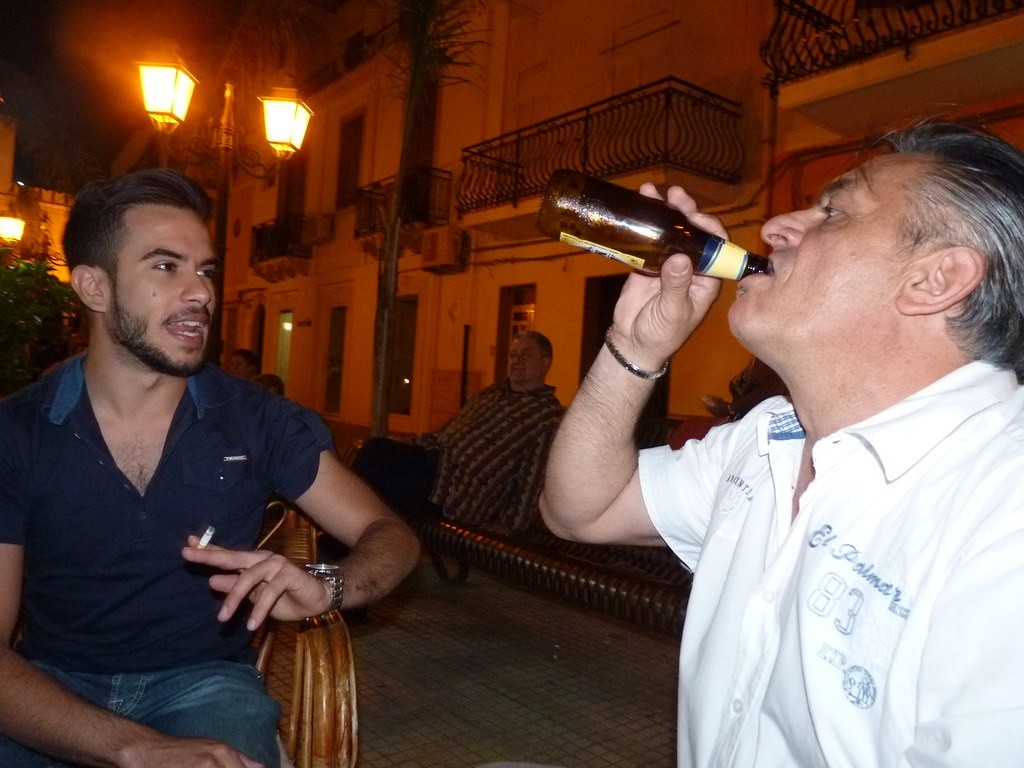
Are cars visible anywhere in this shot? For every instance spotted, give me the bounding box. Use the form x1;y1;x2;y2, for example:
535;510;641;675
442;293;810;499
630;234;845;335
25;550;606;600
51;316;79;345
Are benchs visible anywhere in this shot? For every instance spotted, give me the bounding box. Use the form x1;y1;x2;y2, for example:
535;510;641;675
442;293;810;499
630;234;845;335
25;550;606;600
341;416;693;642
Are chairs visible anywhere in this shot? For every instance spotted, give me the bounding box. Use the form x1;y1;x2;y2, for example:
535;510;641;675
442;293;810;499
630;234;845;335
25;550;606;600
249;499;359;768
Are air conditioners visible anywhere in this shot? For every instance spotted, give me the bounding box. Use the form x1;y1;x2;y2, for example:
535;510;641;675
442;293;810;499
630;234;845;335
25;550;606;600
422;227;466;271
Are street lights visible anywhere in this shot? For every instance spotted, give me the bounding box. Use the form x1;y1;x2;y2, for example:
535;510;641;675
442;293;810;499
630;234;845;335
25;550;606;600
132;43;316;366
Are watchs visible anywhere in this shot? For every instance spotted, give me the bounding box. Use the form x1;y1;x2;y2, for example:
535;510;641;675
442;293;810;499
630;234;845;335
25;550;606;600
303;563;344;611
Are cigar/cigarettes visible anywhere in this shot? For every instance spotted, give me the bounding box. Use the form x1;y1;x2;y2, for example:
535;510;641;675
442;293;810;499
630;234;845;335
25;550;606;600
195;526;215;549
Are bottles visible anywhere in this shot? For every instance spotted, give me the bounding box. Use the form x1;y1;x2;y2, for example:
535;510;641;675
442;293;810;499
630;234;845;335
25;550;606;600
537;169;774;280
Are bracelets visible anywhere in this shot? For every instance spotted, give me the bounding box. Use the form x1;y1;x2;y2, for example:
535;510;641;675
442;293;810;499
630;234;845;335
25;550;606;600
728;405;735;416
607;329;668;379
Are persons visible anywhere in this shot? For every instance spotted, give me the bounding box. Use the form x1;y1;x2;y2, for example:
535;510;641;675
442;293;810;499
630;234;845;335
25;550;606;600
229;348;285;397
539;122;1024;768
706;354;792;424
1;165;421;767
314;329;560;623
